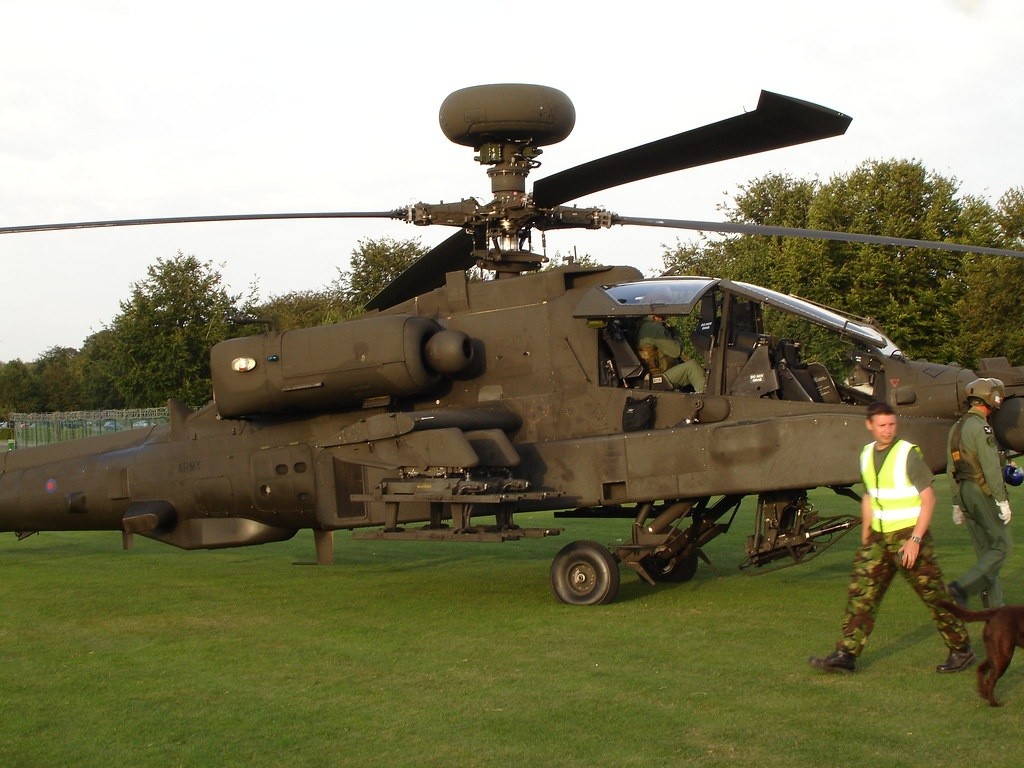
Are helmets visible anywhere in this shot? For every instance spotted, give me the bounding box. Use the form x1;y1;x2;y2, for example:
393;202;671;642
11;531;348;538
963;378;1005;409
1004;464;1024;486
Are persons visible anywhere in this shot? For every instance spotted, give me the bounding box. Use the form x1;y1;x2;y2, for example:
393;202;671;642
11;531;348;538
809;403;977;674
945;378;1012;613
636;314;706;393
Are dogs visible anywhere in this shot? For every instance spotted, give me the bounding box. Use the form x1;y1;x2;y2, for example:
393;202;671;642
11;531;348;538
933;600;1024;708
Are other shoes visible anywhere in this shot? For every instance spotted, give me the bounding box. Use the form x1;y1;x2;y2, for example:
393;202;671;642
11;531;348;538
948;581;968;607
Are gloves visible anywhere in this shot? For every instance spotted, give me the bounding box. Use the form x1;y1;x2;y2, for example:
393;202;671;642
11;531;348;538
996;500;1012;525
952;505;964;525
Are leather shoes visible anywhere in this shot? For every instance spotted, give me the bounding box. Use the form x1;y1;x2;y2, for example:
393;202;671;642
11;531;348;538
937;647;976;672
810;648;856;675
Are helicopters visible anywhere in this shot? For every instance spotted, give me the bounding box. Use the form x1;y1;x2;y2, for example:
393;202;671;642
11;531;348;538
0;80;1024;608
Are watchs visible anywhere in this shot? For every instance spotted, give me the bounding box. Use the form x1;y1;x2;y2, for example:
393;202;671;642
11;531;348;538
908;534;923;544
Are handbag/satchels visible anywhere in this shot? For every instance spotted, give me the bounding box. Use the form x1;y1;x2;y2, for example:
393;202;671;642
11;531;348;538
622;395;656;433
